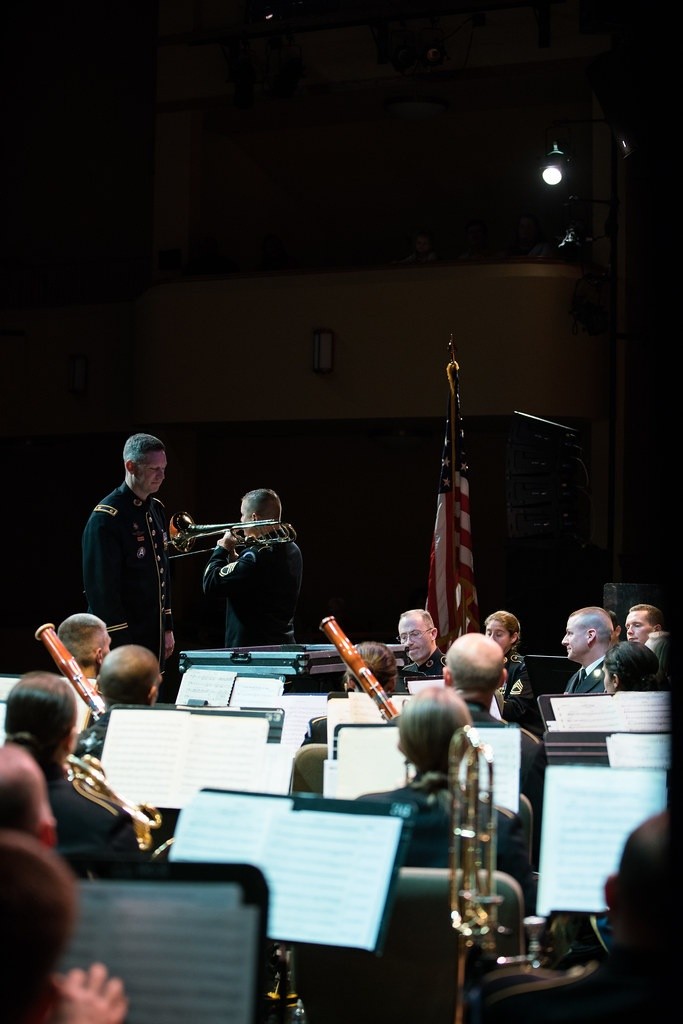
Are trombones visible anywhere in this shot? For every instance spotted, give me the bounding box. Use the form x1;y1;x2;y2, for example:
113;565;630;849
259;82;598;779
442;723;502;1023
167;510;298;560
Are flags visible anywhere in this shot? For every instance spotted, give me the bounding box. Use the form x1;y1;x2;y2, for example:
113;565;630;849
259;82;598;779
424;362;480;654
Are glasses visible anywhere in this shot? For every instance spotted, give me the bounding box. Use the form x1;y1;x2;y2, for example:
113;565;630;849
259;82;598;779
396;628;433;644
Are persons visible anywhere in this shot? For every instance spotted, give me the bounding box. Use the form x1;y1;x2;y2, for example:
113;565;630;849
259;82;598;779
353;685;510;872
396;609;446;674
561;604;671;694
74;644;162;756
441;633;545;804
82;433;175;673
0;671;153;1024
203;490;302;648
464;811;671;1024
303;641;398;745
57;612;112;678
484;610;533;724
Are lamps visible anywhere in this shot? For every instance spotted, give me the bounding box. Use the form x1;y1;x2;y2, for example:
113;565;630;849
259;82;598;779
67;343;104;406
313;326;334;376
264;8;274;25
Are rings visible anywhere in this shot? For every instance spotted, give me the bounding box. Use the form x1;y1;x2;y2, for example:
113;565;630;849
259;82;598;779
171;650;173;652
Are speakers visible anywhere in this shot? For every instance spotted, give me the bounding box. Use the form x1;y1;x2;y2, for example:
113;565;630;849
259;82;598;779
603;583;649;643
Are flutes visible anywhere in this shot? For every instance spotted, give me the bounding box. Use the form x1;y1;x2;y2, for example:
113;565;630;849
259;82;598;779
316;611;399;722
33;622;106;721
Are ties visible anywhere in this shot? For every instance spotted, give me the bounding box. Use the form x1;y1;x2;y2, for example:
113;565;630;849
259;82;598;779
573;668;587;693
